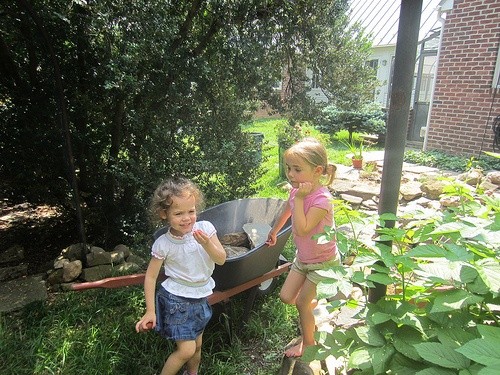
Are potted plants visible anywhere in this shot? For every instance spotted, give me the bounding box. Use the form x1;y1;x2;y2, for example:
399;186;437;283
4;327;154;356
340;137;364;167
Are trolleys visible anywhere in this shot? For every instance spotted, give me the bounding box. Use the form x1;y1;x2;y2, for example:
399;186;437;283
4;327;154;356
75;195;294;311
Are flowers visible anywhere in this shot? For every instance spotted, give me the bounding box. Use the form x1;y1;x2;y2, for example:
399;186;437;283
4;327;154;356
277;123;309;150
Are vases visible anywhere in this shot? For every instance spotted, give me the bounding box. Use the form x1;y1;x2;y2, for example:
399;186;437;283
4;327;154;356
278;147;290;179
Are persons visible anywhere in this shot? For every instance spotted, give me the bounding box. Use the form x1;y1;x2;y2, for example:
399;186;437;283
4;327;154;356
135;178;226;375
265;139;341;357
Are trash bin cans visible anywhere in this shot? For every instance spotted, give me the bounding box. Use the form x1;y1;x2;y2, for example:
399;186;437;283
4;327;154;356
244;132;264;164
